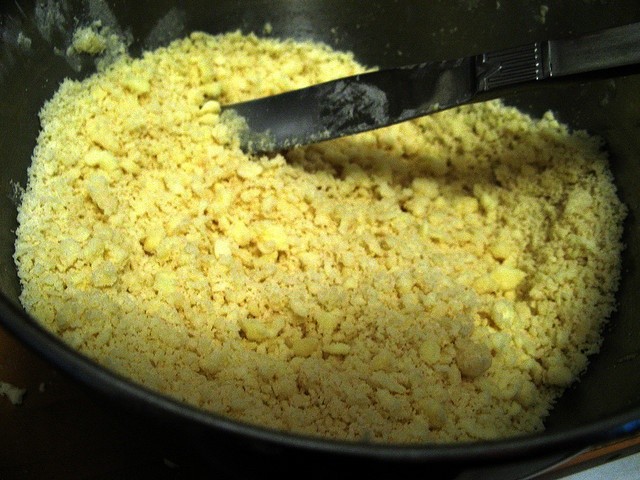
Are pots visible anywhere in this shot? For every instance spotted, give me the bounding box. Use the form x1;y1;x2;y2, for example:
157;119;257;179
1;2;640;457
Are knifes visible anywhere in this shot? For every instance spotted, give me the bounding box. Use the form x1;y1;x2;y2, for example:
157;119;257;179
222;22;640;153
